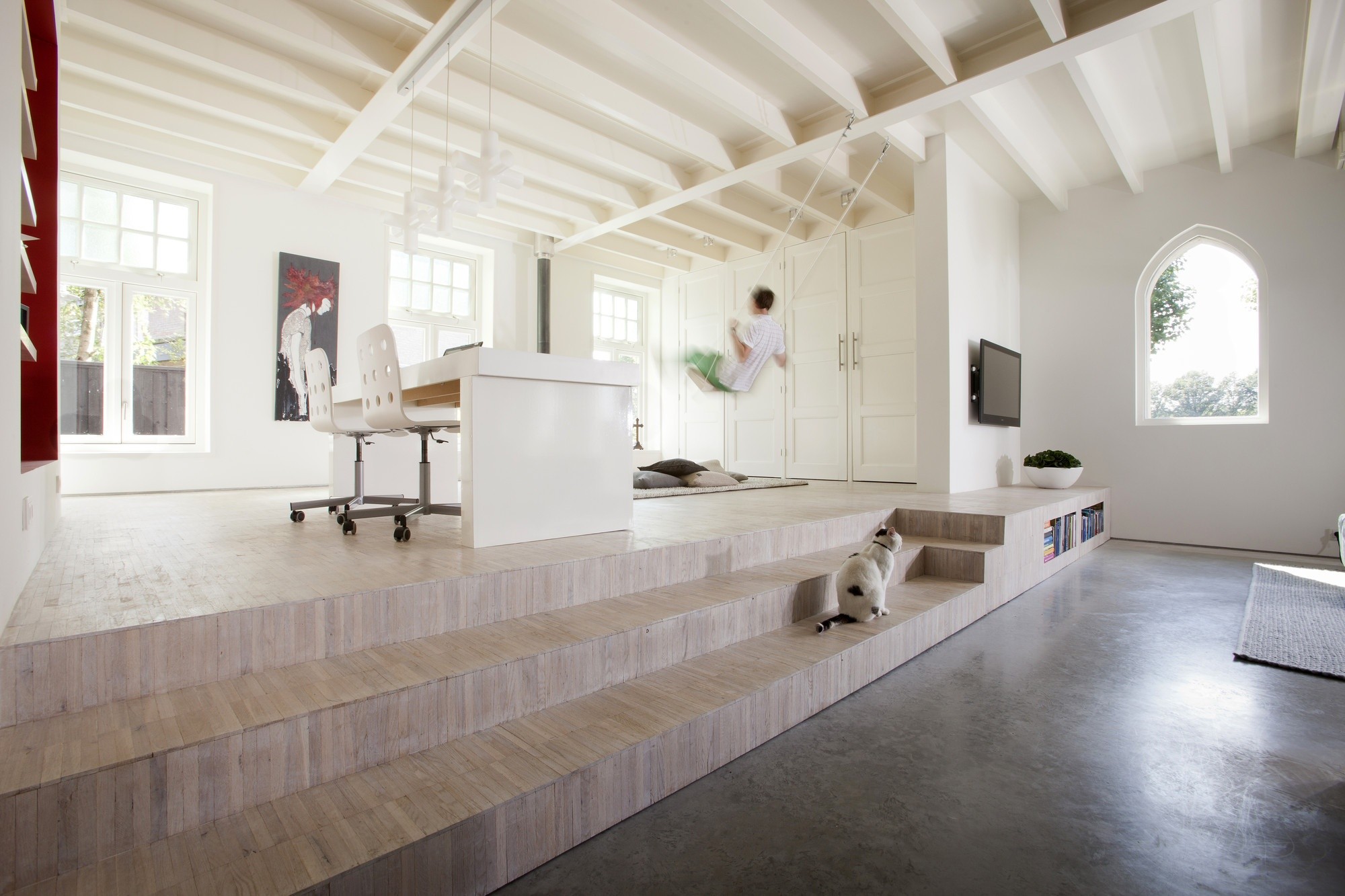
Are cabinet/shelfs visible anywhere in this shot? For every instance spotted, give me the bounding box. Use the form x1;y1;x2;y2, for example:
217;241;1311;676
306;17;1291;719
670;252;786;475
3;1;62;478
790;239;917;484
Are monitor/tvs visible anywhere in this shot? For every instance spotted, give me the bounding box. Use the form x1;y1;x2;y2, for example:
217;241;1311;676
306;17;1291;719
970;338;1021;427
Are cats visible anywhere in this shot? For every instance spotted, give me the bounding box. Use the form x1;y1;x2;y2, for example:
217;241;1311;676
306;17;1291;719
816;521;902;633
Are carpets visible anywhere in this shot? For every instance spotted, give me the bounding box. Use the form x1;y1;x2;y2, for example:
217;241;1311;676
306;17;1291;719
1231;560;1345;679
633;478;809;499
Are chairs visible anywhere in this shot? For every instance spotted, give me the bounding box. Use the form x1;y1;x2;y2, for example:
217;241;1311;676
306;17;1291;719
342;324;461;543
288;348;421;524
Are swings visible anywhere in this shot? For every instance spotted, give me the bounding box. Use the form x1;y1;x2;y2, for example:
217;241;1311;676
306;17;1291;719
686;112;890;393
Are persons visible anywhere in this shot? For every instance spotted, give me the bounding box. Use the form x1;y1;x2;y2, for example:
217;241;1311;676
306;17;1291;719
686;288;789;395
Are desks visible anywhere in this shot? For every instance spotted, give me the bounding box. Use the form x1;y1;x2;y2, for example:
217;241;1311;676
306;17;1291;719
329;345;640;549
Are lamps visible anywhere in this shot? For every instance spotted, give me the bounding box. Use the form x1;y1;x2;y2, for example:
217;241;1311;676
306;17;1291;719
399;0;522;246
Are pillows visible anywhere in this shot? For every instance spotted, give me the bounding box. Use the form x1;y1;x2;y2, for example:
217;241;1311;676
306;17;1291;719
633;471;688;488
637;458;710;477
679;471;740;487
725;472;749;482
697;459;730;477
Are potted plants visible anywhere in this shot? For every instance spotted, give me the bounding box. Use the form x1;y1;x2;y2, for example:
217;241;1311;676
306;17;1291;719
1023;449;1084;490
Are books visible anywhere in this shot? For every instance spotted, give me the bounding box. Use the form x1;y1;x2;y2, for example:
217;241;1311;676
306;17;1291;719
1044;508;1105;564
